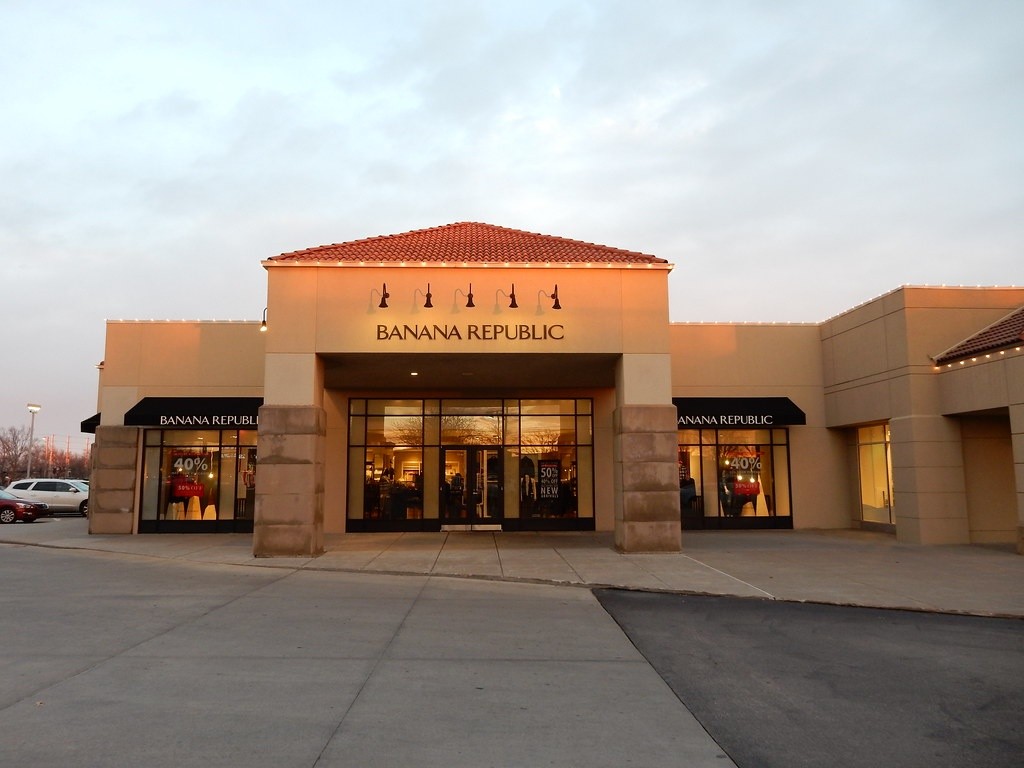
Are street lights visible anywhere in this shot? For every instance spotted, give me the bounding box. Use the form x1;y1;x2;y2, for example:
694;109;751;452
25;402;42;479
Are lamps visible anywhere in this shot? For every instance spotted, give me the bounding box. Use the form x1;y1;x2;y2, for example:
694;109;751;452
378;283;390;308
551;284;561;309
466;283;475;307
422;284;434;308
259;307;267;331
508;283;519;309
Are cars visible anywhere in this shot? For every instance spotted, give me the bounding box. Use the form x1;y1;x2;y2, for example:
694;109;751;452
0;486;44;524
3;478;89;517
